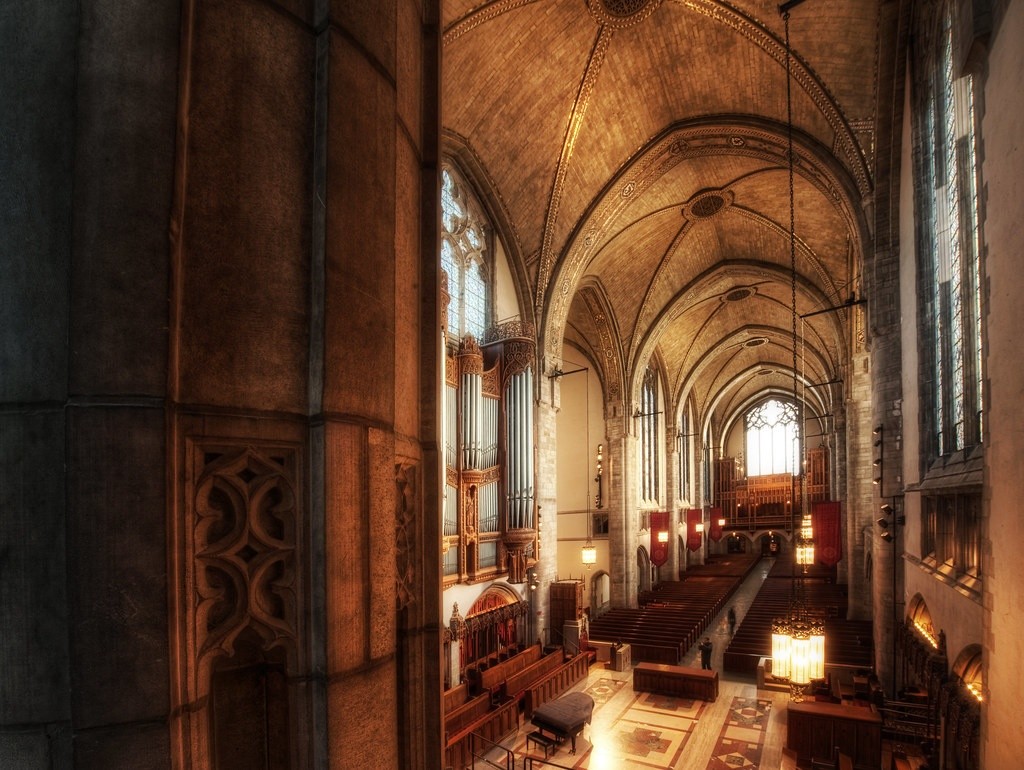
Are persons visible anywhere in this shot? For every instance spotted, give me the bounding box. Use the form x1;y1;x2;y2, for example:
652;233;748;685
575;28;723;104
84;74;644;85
727;607;736;638
698;636;713;670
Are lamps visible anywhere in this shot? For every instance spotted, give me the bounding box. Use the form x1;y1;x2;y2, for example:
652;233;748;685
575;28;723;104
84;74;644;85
872;423;884;498
793;511;817;574
875;518;894;528
769;591;827;703
582;495;596;570
696;520;704;536
718;516;725;529
881;504;894;516
881;532;894;543
658;530;669;550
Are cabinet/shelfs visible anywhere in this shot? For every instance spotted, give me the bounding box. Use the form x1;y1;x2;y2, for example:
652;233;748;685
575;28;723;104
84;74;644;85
783;696;882;770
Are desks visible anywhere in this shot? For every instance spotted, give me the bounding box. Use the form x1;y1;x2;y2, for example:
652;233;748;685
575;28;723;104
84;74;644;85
851;672;870;696
632;661;719;702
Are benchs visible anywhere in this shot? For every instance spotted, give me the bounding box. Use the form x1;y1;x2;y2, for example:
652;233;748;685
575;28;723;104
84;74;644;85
527;731;557;758
836;678;853;699
444;644;568;744
589;550;764;667
723;577;876;681
